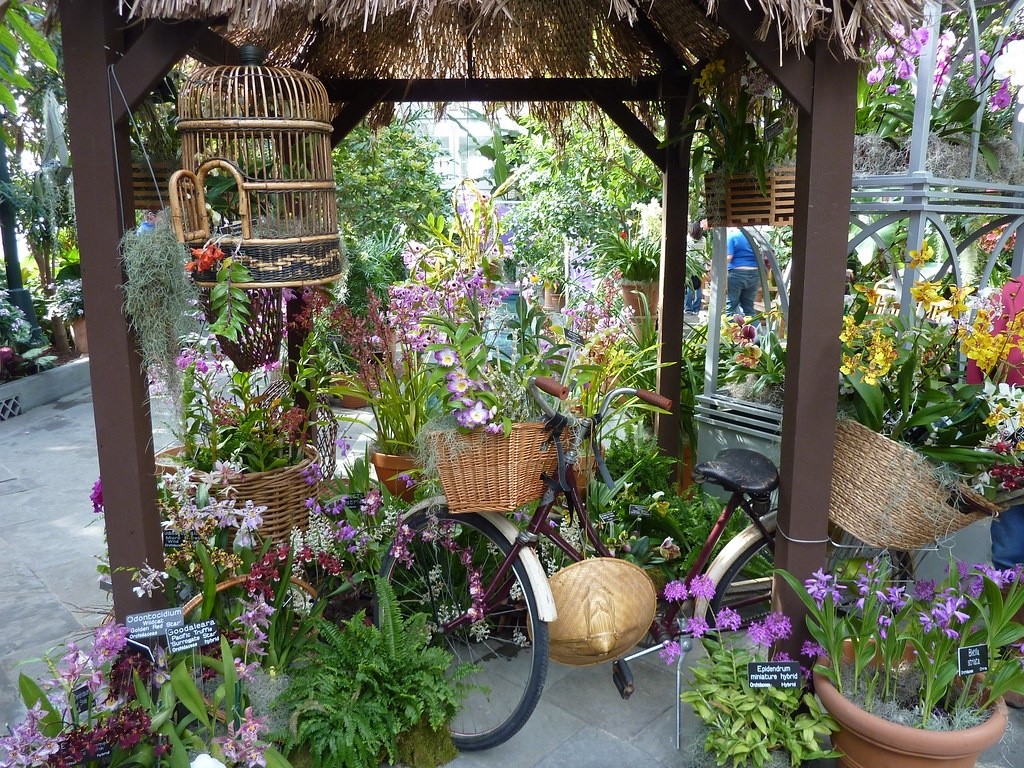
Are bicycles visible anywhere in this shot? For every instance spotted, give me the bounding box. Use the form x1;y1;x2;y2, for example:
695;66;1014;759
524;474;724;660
374;372;919;754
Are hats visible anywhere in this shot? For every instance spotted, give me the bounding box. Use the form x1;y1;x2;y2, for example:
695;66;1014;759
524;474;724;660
526;557;658;666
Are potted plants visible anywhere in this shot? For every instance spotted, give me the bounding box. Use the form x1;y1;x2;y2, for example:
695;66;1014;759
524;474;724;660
569;209;661;315
128;98;183;210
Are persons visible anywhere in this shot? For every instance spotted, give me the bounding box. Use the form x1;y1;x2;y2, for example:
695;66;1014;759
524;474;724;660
683;221;707;315
136;209;158;240
967;276;1024;589
727;224;761;317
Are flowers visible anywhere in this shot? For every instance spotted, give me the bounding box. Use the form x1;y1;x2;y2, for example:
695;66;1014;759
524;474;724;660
0;20;1024;768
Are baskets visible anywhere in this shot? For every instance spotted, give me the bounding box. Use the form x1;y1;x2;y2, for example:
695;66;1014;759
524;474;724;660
827;414;1010;551
427;421;580;515
153;441;327;554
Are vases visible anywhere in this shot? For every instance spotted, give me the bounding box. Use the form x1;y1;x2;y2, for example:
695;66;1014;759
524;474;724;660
180;574;323;636
543;282;554;311
808;635;1008;767
367;352;383;366
628;314;658;343
1001;647;1023;709
333;377;367;408
571;440;606;503
999;582;1023;626
702;163;794;229
370;451;423;507
550;293;565;313
68;316;88;354
